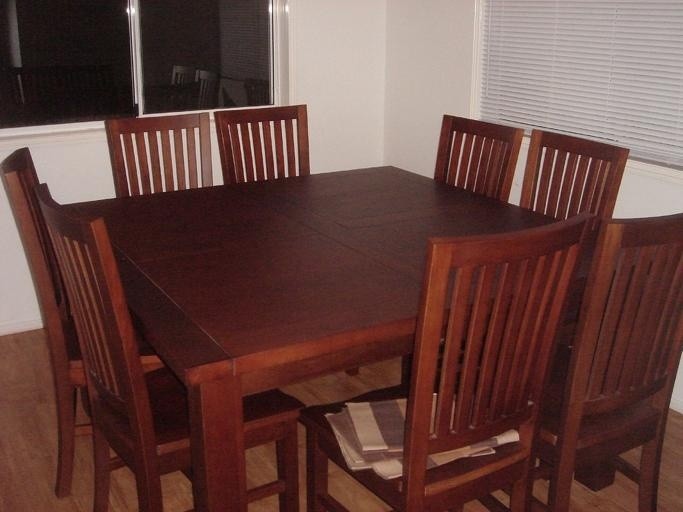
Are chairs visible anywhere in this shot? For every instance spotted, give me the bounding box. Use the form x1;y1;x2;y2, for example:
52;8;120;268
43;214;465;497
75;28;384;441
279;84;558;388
4;53;270;122
